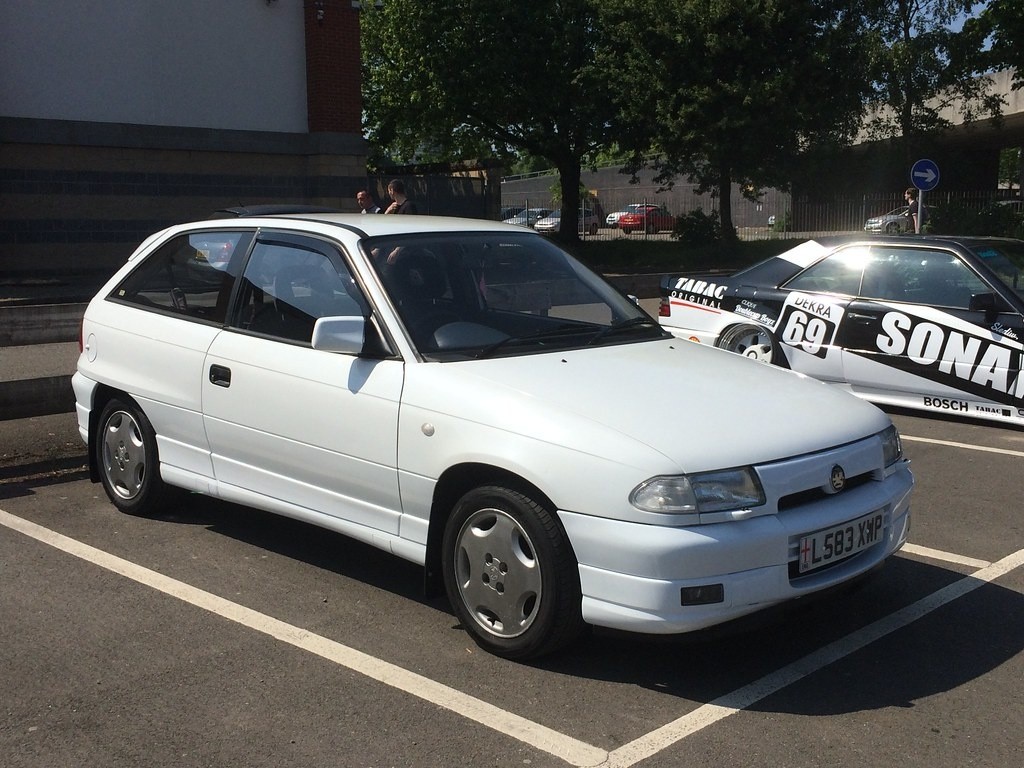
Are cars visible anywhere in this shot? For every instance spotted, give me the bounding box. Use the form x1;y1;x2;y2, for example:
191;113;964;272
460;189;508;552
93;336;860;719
68;208;917;666
864;202;944;235
768;214;790;227
605;202;674;229
616;204;677;235
533;207;601;236
500;207;555;232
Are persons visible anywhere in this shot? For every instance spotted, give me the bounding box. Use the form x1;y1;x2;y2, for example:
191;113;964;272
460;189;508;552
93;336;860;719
384;179;416;263
904;187;926;234
356;188;384;261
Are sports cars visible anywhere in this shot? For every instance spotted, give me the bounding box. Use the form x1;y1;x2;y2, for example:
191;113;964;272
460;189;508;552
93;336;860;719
657;234;1024;428
